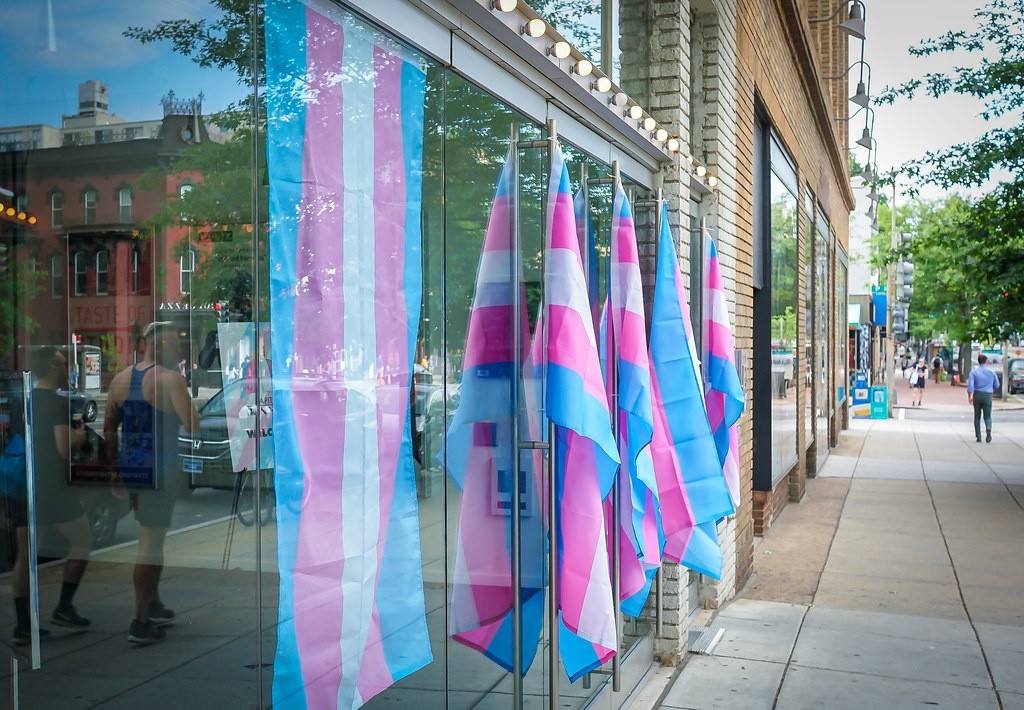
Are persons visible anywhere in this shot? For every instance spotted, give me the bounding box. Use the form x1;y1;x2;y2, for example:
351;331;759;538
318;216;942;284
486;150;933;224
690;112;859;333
9;345;93;646
930;354;944;384
421;354;434;373
103;321;200;643
177;358;249;398
900;355;929;406
967;355;1000;442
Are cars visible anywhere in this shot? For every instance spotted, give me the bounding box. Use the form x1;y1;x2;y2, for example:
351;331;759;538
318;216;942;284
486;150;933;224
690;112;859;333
365;385;461;457
0;373;99;422
1009;357;1024;394
394;364;434;383
177;376;382;494
0;420;136;561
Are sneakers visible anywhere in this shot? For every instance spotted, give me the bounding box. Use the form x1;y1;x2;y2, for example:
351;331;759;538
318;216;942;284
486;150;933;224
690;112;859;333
149;603;176;623
126;618;168;643
11;624;52;642
50;606;93;630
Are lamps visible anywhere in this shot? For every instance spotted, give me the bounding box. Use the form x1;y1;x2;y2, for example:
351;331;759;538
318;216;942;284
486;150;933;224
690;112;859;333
808;0;882;230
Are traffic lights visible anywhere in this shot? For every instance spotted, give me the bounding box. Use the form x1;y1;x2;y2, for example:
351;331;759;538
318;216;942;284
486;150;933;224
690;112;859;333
896;262;915;299
898;232;912;244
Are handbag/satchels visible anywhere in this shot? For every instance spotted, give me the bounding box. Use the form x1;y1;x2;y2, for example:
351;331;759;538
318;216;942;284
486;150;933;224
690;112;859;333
940;370;948;382
909;364;919;385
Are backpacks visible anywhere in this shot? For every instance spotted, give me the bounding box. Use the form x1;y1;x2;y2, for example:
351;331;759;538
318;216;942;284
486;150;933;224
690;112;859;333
934;356;941;368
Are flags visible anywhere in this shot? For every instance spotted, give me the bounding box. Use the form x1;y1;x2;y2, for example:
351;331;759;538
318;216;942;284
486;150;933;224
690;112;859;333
545;132;623;685
610;178;667;619
701;229;745;507
434;143;550;679
648;198;735;579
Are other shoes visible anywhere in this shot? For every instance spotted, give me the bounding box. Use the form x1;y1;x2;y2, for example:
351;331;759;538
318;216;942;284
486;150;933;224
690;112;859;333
913;401;915;406
918;401;921;405
986;433;991;442
977;437;981;442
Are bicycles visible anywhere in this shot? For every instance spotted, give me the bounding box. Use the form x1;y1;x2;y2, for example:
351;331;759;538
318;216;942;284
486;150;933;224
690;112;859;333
236;415;314;528
416;408;456;474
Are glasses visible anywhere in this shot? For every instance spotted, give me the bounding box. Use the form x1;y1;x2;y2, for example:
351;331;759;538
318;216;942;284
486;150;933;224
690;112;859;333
157;340;179;348
53;361;70;369
